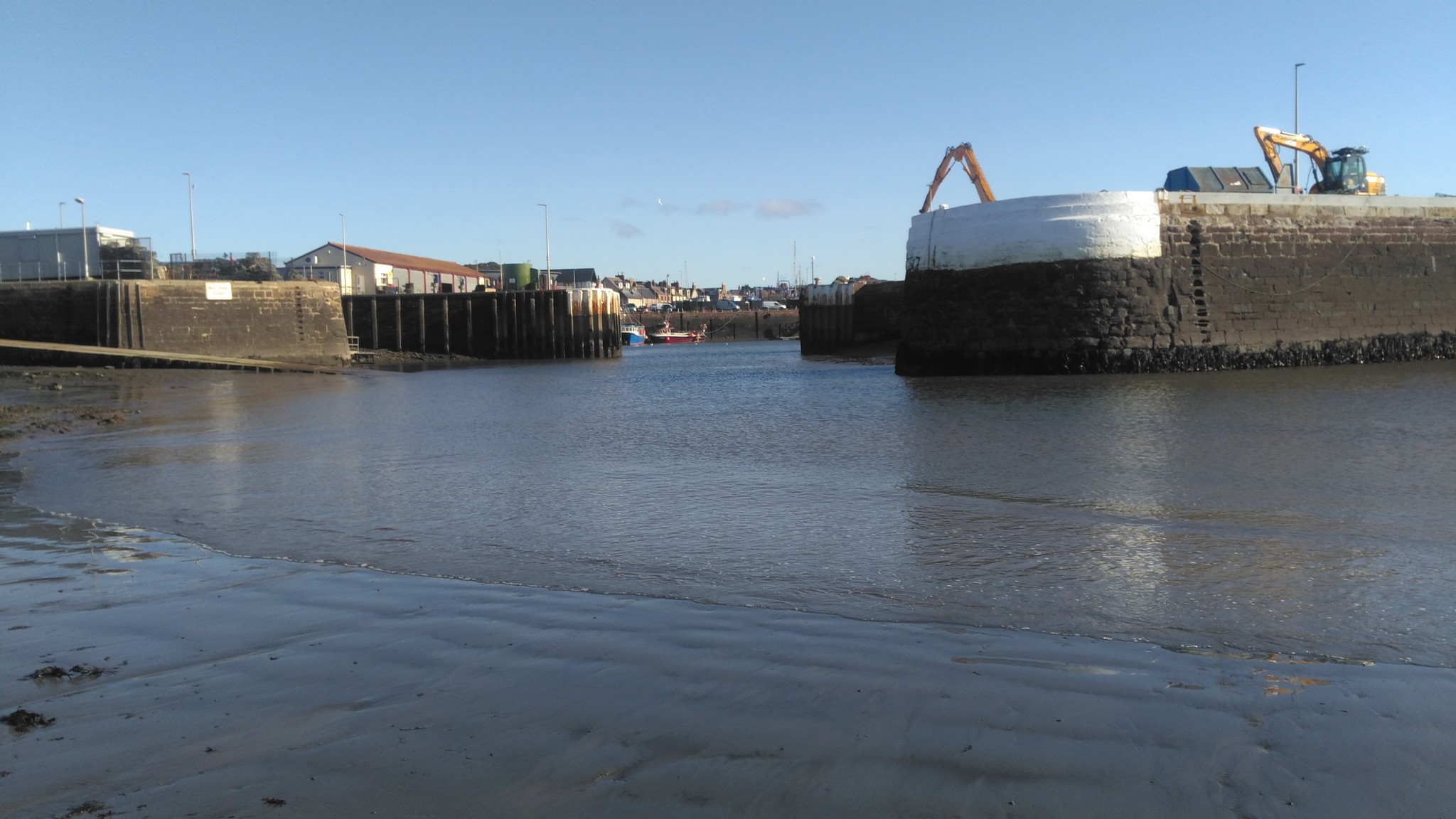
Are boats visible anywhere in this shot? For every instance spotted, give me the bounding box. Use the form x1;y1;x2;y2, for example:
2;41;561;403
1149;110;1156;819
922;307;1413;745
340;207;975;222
648;321;707;344
620;304;646;346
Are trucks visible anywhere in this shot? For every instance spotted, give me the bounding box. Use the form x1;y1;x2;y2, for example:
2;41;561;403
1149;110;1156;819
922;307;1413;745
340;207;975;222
649;303;676;314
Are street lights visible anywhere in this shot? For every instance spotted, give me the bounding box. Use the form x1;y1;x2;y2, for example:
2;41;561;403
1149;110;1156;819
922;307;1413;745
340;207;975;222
811;256;815;284
181;172;196;261
1295;62;1307;185
74;196;89;280
537;204;551;290
339;213;347;296
679;271;684;312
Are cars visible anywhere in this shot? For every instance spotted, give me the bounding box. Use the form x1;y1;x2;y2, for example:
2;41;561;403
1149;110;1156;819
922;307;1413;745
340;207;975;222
660;304;673;314
638;306;649;314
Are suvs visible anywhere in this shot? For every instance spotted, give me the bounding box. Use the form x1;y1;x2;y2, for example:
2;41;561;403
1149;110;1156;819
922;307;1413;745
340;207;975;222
624;303;637;314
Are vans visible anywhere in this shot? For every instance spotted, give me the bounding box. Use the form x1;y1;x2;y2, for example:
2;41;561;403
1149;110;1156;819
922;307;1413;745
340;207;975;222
762;301;786;310
715;301;740;312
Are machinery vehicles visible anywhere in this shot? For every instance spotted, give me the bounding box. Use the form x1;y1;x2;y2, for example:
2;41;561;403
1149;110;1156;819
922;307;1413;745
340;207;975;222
919;142;995;212
1253;124;1386;197
